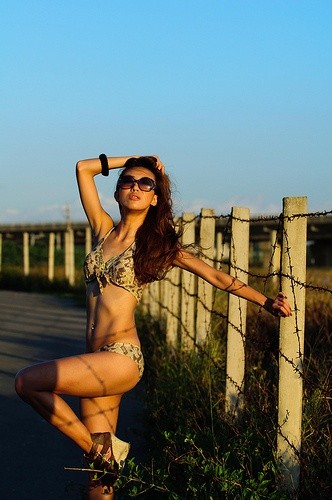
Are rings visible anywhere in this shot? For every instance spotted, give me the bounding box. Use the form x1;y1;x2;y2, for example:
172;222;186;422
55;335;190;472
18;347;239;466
280;302;283;306
278;306;281;310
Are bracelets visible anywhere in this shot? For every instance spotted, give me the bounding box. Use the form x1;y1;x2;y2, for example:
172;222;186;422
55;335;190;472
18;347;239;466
98;154;110;177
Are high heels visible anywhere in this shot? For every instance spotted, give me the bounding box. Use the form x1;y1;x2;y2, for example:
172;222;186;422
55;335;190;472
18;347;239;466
86;430;131;494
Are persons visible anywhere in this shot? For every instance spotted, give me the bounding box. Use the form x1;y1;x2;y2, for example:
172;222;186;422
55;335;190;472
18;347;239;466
14;155;293;500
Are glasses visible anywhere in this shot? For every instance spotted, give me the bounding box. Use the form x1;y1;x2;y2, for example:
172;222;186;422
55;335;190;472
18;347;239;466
117;175;158;194
